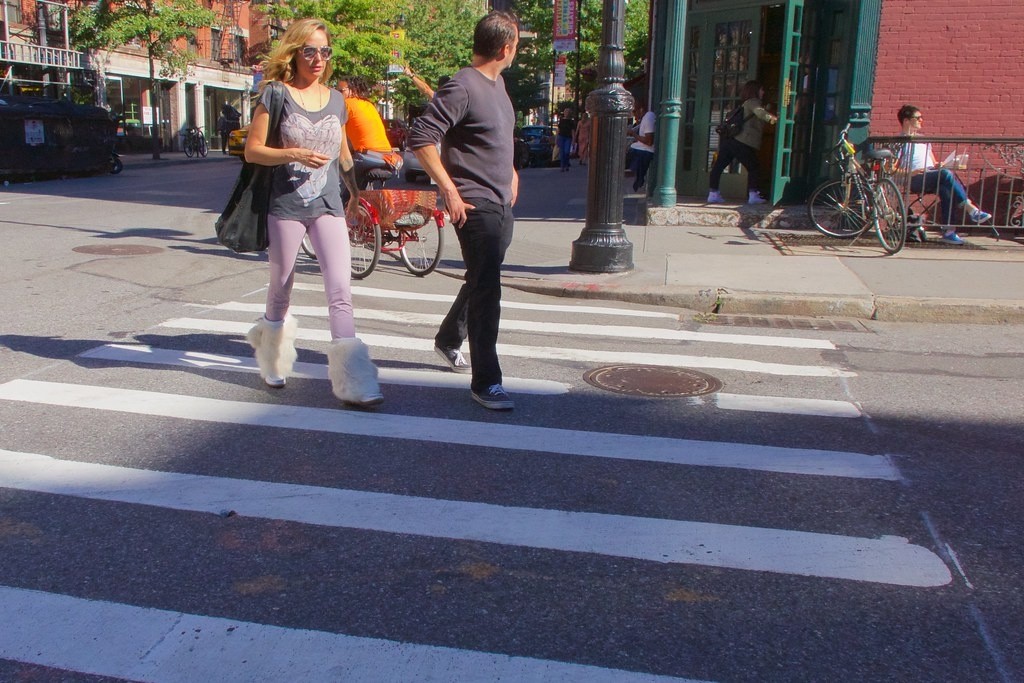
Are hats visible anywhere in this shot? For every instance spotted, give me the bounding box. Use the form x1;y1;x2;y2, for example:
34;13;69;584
337;81;349;88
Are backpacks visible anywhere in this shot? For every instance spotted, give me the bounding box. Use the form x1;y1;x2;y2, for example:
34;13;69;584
716;97;763;138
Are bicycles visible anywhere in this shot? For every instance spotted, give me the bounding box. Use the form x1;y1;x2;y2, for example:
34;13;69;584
808;124;906;253
180;127;209;157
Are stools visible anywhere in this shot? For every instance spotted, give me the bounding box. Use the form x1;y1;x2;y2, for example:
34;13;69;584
906;190;941;223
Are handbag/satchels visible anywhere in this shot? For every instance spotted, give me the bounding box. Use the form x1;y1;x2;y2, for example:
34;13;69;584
215;80;285;253
570;143;577;153
552;145;560;161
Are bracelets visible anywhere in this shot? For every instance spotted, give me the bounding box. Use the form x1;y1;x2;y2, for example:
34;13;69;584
410;74;415;79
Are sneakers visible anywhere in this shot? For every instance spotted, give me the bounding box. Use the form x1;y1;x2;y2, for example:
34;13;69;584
707;191;725;203
971;210;991;223
943;233;964;244
748;191;766;204
434;344;472;374
470;384;515;409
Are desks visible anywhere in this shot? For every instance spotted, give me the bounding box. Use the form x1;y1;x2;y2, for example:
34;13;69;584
943;167;1009;239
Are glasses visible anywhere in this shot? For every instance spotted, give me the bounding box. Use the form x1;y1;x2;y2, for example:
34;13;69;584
906;116;922;120
297;46;332;61
632;106;639;114
338;89;346;93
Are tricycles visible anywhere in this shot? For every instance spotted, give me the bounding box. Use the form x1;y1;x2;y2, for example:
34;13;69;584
301;166;445;279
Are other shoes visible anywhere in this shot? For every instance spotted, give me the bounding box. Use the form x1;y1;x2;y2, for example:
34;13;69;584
579;160;586;165
561;168;569;172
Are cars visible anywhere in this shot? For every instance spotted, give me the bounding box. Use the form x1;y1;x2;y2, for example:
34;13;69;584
514;129;533;167
404;146;429;183
382;118;410;149
520;125;559;161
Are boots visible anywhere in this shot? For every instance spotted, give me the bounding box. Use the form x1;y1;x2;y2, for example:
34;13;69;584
248;314;300;387
326;337;384;405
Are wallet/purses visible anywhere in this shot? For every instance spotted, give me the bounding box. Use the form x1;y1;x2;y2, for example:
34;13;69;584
363;148;404;176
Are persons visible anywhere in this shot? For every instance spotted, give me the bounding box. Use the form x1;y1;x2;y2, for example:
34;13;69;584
405;67;451;199
335;77;404;206
244;20;385;407
625;104;657;192
554;107;591;172
707;80;778;205
219;104;240;155
888;104;993;245
409;11;519;411
